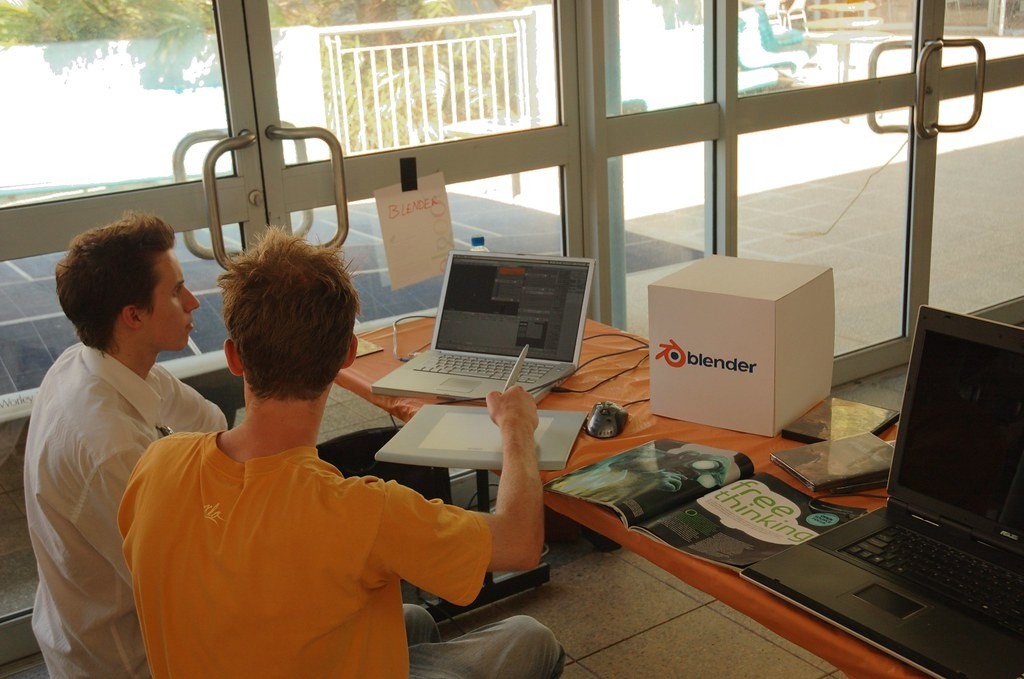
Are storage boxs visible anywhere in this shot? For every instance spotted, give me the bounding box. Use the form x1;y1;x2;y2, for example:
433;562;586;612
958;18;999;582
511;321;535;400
648;256;835;437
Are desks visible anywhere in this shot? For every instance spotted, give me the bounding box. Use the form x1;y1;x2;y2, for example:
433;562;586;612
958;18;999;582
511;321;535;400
804;1;892;84
335;316;936;679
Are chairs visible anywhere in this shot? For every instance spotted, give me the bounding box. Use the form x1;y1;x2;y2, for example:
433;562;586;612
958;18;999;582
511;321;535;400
738;7;809;96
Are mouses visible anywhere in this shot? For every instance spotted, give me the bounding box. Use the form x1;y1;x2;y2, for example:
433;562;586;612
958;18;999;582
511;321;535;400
586;400;629;439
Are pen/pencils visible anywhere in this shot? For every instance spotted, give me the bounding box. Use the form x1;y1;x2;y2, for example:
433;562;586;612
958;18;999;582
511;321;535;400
500;343;530;395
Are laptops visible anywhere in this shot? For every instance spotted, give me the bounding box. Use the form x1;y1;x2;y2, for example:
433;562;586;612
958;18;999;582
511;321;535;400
372;249;597;405
737;304;1024;678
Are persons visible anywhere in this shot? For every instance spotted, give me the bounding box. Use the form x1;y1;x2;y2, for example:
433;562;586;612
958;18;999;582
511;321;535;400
118;228;567;679
22;219;229;679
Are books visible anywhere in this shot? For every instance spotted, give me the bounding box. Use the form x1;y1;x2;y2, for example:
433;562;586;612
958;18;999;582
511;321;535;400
543;437;871;576
